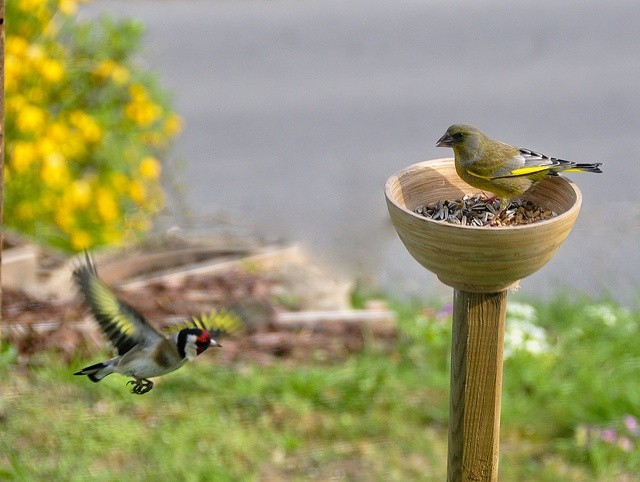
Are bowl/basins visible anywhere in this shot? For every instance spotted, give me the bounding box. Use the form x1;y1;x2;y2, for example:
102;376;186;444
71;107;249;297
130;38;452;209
383;156;582;295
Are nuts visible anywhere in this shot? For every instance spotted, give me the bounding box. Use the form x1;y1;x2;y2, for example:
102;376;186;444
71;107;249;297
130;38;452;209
407;196;522;231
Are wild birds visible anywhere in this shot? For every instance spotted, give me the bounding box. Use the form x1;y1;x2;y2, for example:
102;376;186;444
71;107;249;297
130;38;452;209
73;244;246;395
436;124;603;226
